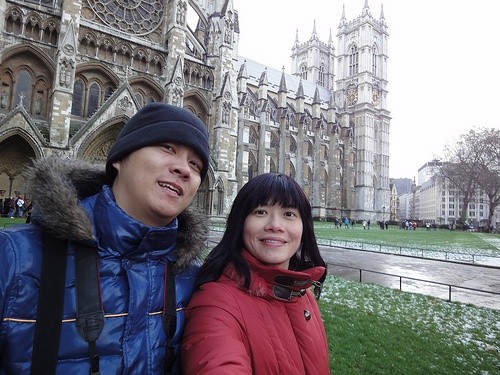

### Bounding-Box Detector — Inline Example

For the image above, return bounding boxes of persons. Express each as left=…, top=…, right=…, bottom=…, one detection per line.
left=334, top=217, right=494, bottom=233
left=0, top=195, right=32, bottom=223
left=0, top=103, right=210, bottom=375
left=181, top=172, right=330, bottom=375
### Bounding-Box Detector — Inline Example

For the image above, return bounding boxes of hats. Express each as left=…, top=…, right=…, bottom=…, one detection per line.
left=106, top=101, right=211, bottom=186
